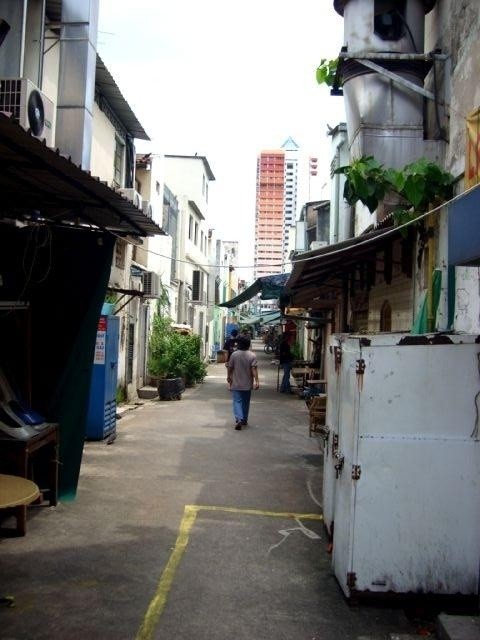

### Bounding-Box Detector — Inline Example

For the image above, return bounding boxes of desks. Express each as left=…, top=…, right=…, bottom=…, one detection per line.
left=0, top=422, right=62, bottom=537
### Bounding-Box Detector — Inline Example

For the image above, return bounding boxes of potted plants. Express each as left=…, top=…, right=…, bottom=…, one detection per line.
left=147, top=310, right=202, bottom=401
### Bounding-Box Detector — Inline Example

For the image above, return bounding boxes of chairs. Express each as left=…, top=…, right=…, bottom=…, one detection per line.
left=305, top=394, right=326, bottom=437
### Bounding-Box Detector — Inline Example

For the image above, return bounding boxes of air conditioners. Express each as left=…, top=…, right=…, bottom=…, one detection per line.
left=0, top=77, right=57, bottom=148
left=142, top=271, right=160, bottom=298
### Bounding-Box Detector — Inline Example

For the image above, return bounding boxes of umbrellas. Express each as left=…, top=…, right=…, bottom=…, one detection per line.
left=411, top=266, right=444, bottom=335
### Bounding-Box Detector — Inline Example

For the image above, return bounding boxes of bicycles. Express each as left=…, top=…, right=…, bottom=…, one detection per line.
left=264, top=338, right=279, bottom=354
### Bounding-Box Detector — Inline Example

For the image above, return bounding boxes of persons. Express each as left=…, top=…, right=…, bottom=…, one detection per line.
left=223, top=328, right=240, bottom=390
left=307, top=336, right=322, bottom=380
left=226, top=335, right=260, bottom=431
left=277, top=329, right=297, bottom=394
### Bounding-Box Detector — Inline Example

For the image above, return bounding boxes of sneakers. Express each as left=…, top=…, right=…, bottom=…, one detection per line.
left=236, top=418, right=249, bottom=431
